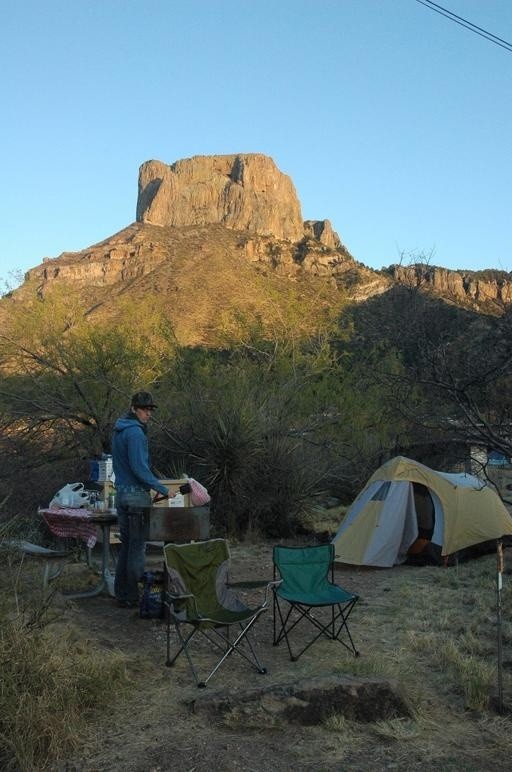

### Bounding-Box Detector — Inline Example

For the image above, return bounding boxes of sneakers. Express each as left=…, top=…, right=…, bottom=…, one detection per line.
left=119, top=598, right=141, bottom=609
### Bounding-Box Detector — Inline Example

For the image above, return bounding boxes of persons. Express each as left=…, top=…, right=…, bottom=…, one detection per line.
left=110, top=391, right=177, bottom=609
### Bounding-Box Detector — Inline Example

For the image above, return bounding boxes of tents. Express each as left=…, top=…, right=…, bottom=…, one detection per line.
left=330, top=455, right=512, bottom=568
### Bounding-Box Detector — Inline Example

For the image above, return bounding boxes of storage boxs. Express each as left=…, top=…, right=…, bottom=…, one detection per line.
left=88, top=459, right=194, bottom=544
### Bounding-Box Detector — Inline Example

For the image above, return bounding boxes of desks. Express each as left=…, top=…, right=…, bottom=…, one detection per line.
left=38, top=508, right=119, bottom=600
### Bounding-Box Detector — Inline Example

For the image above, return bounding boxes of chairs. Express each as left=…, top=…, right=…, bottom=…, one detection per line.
left=163, top=539, right=282, bottom=688
left=271, top=544, right=360, bottom=662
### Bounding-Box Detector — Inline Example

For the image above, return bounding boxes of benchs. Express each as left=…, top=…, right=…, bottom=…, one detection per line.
left=2, top=538, right=69, bottom=599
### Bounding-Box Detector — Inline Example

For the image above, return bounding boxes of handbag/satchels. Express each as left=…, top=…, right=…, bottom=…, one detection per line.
left=142, top=570, right=167, bottom=619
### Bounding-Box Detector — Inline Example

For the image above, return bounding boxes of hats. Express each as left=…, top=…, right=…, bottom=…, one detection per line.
left=131, top=392, right=158, bottom=408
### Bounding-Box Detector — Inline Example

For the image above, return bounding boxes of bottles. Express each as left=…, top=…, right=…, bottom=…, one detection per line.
left=109, top=488, right=116, bottom=508
